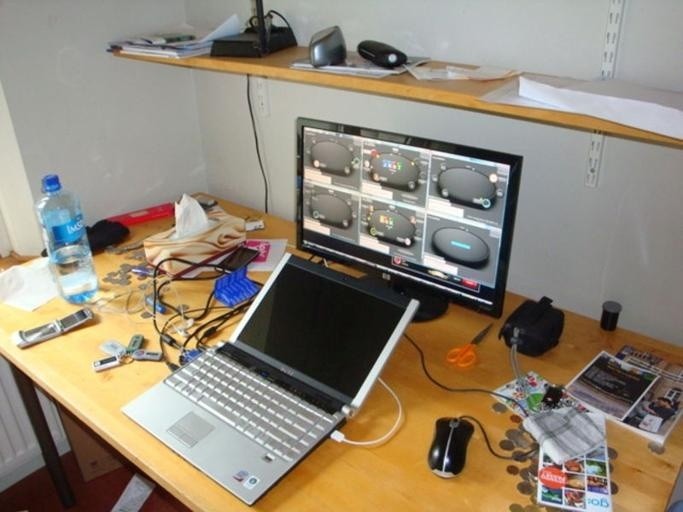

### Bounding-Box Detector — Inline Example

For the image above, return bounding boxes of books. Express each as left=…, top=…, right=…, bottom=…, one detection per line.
left=490, top=341, right=683, bottom=512
left=292, top=49, right=432, bottom=76
left=110, top=13, right=247, bottom=61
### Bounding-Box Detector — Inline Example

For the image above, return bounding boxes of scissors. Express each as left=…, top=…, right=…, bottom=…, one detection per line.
left=446, top=324, right=493, bottom=367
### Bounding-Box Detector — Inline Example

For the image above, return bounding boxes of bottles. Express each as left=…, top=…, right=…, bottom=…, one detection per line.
left=601, top=300, right=622, bottom=330
left=33, top=173, right=99, bottom=301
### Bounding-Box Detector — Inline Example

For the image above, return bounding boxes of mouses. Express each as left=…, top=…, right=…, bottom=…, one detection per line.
left=428, top=417, right=473, bottom=478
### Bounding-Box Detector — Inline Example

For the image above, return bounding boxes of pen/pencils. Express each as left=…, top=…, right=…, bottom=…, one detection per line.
left=146, top=296, right=166, bottom=314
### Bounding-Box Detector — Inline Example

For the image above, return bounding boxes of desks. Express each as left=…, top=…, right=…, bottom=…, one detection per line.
left=0, top=191, right=683, bottom=511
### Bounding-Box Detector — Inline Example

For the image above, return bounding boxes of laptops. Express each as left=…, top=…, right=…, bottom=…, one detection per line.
left=120, top=252, right=420, bottom=507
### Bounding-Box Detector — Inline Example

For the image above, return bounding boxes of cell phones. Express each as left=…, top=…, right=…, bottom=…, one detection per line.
left=215, top=245, right=259, bottom=275
left=15, top=307, right=94, bottom=349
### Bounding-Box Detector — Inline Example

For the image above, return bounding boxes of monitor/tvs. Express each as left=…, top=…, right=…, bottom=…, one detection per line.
left=296, top=116, right=522, bottom=322
